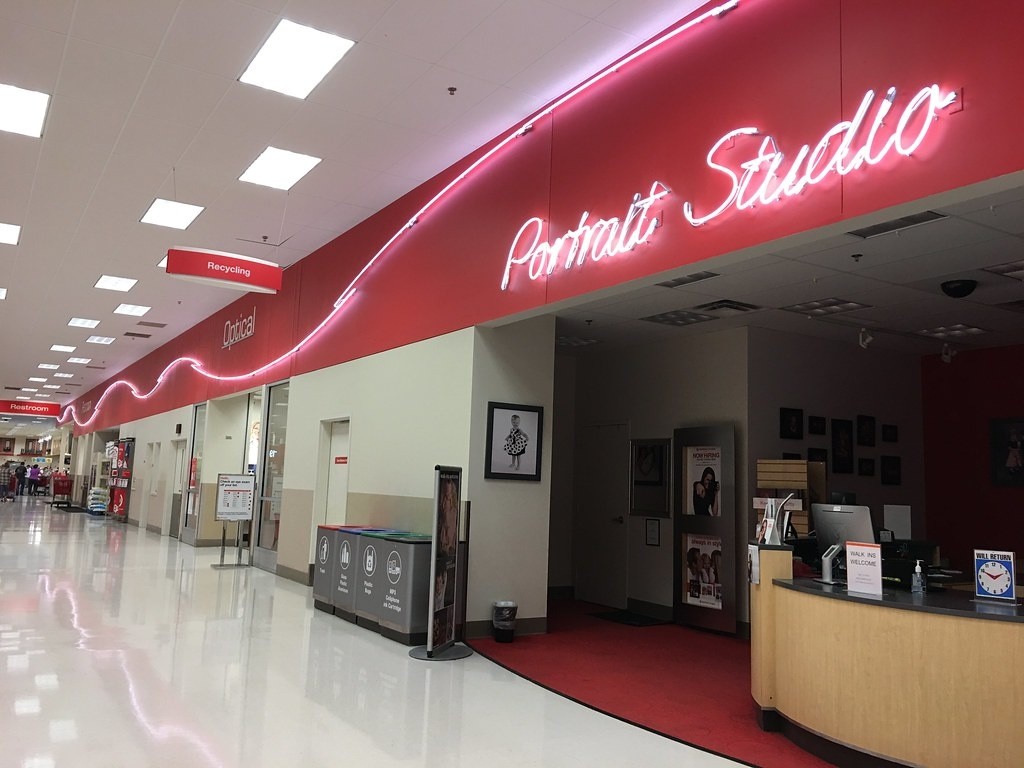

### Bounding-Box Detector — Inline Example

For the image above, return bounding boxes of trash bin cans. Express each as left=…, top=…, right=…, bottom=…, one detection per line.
left=492, top=600, right=519, bottom=644
left=354, top=532, right=410, bottom=634
left=334, top=528, right=409, bottom=625
left=313, top=524, right=384, bottom=616
left=380, top=537, right=467, bottom=647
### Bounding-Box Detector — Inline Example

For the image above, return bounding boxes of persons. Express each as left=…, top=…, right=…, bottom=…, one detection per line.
left=28, top=464, right=42, bottom=496
left=693, top=467, right=718, bottom=516
left=504, top=415, right=529, bottom=470
left=15, top=462, right=27, bottom=495
left=25, top=464, right=32, bottom=488
left=0, top=463, right=11, bottom=502
left=432, top=477, right=459, bottom=611
left=687, top=548, right=721, bottom=599
left=248, top=422, right=261, bottom=476
left=37, top=466, right=70, bottom=478
left=757, top=520, right=767, bottom=544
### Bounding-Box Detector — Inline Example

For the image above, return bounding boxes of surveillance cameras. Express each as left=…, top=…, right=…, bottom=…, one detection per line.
left=942, top=279, right=978, bottom=298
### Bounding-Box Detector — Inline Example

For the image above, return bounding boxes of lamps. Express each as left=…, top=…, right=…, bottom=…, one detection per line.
left=856, top=332, right=875, bottom=352
left=942, top=343, right=958, bottom=363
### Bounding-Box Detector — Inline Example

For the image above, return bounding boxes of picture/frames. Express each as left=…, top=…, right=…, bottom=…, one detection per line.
left=880, top=455, right=901, bottom=486
left=989, top=417, right=1024, bottom=487
left=830, top=418, right=854, bottom=474
left=882, top=424, right=898, bottom=443
left=858, top=457, right=875, bottom=476
left=808, top=415, right=826, bottom=435
left=807, top=447, right=828, bottom=481
left=631, top=437, right=673, bottom=518
left=635, top=445, right=664, bottom=485
left=857, top=415, right=876, bottom=447
left=782, top=453, right=802, bottom=461
left=484, top=402, right=543, bottom=482
left=780, top=407, right=803, bottom=440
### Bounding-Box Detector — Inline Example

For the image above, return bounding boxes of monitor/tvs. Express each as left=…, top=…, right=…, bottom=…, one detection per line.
left=811, top=504, right=875, bottom=585
